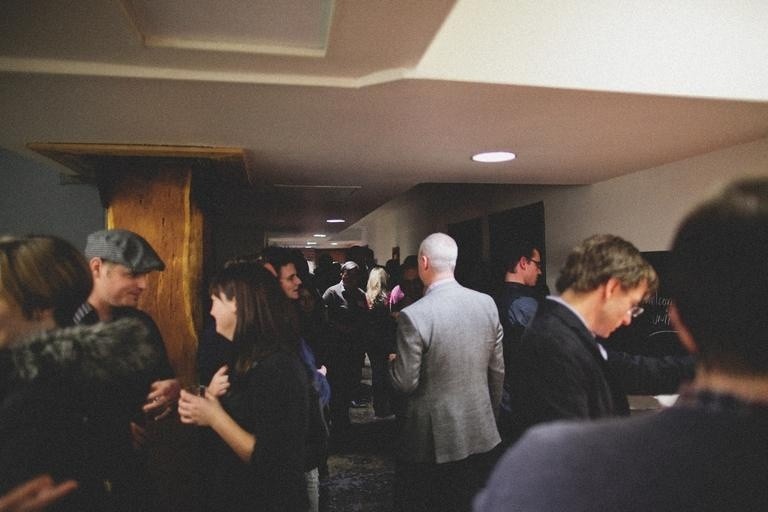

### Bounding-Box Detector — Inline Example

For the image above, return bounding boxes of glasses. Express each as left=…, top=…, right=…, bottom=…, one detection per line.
left=624, top=286, right=645, bottom=318
left=527, top=257, right=541, bottom=269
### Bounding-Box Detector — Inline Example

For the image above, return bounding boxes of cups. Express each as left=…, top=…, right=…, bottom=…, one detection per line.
left=184, top=384, right=209, bottom=402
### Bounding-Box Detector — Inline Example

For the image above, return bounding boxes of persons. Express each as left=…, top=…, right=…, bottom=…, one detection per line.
left=178, top=232, right=505, bottom=512
left=1, top=230, right=184, bottom=512
left=472, top=176, right=768, bottom=512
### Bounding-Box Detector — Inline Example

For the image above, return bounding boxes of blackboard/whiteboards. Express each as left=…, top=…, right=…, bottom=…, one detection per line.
left=596, top=250, right=691, bottom=359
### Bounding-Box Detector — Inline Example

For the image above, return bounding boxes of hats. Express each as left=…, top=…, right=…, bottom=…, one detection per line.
left=340, top=261, right=360, bottom=271
left=84, top=229, right=165, bottom=273
